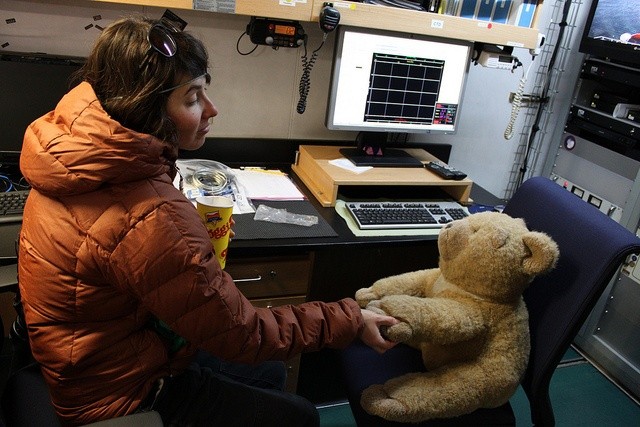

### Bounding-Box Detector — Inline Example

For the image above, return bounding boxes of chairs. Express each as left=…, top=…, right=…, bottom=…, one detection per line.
left=340, top=175, right=640, bottom=426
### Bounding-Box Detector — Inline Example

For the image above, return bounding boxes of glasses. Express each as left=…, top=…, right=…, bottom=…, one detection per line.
left=138, top=17, right=182, bottom=70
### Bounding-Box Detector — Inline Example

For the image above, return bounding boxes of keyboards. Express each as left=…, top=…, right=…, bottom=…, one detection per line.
left=345, top=200, right=470, bottom=229
left=0, top=190, right=30, bottom=223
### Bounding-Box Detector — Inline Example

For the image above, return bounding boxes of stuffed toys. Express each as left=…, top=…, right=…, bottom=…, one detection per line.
left=354, top=211, right=561, bottom=423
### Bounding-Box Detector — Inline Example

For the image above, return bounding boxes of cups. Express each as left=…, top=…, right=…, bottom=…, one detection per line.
left=195, top=195, right=233, bottom=269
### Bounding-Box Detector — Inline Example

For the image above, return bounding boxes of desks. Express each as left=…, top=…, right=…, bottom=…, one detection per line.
left=175, top=156, right=508, bottom=249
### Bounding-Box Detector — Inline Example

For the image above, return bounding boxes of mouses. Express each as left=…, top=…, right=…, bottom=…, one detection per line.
left=493, top=205, right=505, bottom=211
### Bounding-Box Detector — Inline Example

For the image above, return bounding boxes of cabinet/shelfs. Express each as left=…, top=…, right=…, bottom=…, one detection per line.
left=105, top=0, right=540, bottom=50
left=222, top=250, right=316, bottom=397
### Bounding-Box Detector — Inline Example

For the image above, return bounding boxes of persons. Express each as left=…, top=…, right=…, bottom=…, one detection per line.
left=17, top=16, right=402, bottom=425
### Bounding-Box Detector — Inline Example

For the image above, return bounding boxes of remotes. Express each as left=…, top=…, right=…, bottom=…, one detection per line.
left=424, top=162, right=456, bottom=180
left=432, top=160, right=467, bottom=180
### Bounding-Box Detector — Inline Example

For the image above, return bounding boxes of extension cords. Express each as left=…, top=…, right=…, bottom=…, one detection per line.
left=613, top=103, right=640, bottom=118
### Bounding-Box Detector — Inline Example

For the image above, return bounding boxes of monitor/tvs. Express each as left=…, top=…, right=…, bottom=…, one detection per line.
left=0, top=50, right=89, bottom=157
left=578, top=0, right=639, bottom=64
left=325, top=25, right=474, bottom=167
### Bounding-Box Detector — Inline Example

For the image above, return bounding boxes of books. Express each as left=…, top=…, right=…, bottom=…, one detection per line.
left=359, top=0, right=448, bottom=15
